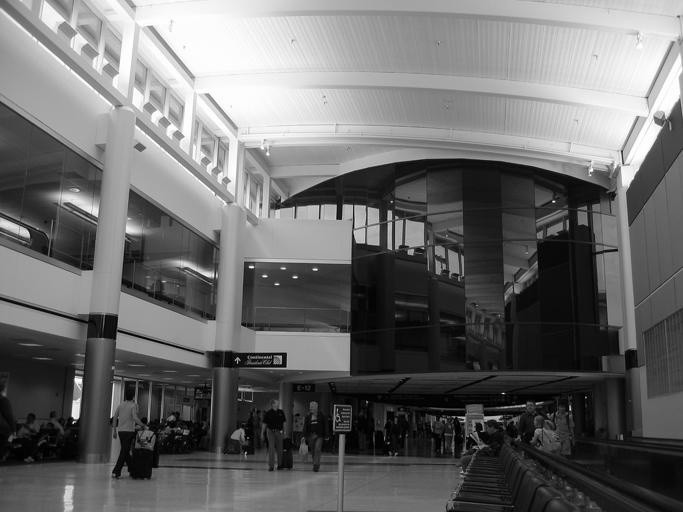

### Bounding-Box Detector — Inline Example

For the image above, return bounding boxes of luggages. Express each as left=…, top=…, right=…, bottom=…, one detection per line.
left=130, top=447, right=154, bottom=480
left=280, top=440, right=294, bottom=468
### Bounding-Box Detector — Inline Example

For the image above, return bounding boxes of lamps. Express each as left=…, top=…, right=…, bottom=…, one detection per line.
left=652, top=109, right=672, bottom=132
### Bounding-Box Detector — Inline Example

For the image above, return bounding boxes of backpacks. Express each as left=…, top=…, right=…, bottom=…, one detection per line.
left=134, top=430, right=155, bottom=450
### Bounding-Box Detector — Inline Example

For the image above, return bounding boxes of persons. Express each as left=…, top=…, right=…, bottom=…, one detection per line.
left=0, top=411, right=80, bottom=463
left=346, top=398, right=576, bottom=472
left=142, top=410, right=209, bottom=455
left=302, top=400, right=330, bottom=472
left=260, top=399, right=287, bottom=472
left=111, top=389, right=149, bottom=479
left=0, top=384, right=17, bottom=462
left=227, top=423, right=250, bottom=455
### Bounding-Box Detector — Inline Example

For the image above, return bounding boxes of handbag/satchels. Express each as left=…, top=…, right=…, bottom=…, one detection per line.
left=299, top=437, right=309, bottom=457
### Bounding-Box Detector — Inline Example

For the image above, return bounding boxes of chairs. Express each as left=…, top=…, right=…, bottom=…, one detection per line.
left=443, top=430, right=682, bottom=511
left=0, top=419, right=79, bottom=461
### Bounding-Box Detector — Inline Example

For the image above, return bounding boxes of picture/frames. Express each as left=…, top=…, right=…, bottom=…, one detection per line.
left=0, top=371, right=9, bottom=398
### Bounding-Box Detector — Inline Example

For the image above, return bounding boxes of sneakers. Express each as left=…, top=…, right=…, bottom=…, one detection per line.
left=455, top=461, right=469, bottom=478
left=268, top=466, right=283, bottom=471
left=110, top=472, right=123, bottom=480
left=387, top=450, right=400, bottom=458
left=312, top=465, right=320, bottom=472
left=23, top=456, right=34, bottom=464
